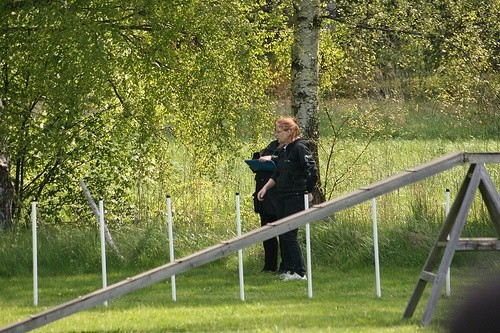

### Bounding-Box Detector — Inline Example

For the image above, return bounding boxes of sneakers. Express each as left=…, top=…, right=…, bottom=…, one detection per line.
left=279, top=270, right=291, bottom=280
left=281, top=271, right=308, bottom=282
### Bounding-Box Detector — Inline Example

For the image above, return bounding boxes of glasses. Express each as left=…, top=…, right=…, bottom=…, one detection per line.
left=275, top=129, right=287, bottom=133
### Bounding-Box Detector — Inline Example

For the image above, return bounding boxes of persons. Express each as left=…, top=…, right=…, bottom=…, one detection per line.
left=258, top=117, right=319, bottom=282
left=245, top=119, right=290, bottom=275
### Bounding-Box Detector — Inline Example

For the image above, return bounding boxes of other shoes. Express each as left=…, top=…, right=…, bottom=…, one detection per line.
left=260, top=269, right=278, bottom=273
